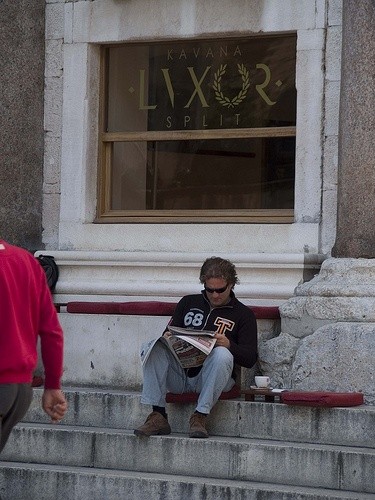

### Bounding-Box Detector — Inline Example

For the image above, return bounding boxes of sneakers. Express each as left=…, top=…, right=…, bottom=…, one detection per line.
left=134, top=412, right=171, bottom=436
left=190, top=413, right=209, bottom=438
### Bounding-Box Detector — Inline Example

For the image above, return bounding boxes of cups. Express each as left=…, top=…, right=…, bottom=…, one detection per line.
left=255, top=375, right=273, bottom=388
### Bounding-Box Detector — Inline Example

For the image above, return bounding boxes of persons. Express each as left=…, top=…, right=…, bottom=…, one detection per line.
left=0, top=237, right=68, bottom=455
left=133, top=256, right=259, bottom=440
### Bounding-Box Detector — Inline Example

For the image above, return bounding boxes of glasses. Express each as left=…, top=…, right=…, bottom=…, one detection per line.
left=204, top=282, right=229, bottom=293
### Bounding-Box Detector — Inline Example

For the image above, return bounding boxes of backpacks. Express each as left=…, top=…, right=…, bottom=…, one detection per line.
left=34, top=255, right=59, bottom=295
